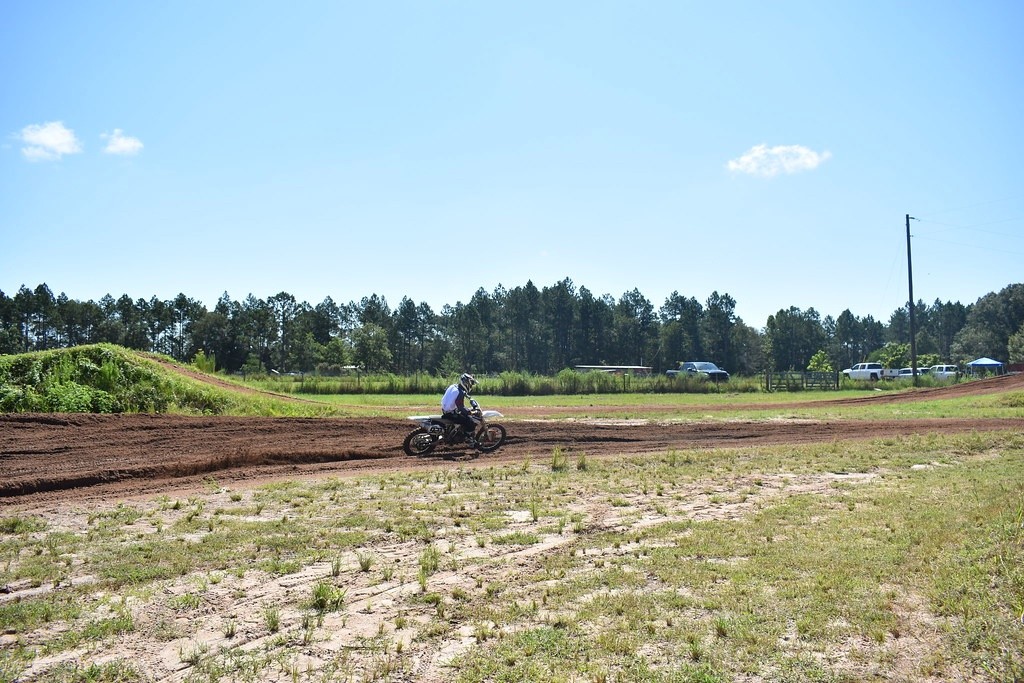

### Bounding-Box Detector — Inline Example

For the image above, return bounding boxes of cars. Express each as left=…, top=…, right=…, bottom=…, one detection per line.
left=899, top=368, right=922, bottom=378
left=930, top=365, right=959, bottom=379
left=918, top=367, right=930, bottom=375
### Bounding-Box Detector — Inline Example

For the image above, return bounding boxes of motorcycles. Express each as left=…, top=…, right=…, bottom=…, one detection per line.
left=403, top=393, right=506, bottom=456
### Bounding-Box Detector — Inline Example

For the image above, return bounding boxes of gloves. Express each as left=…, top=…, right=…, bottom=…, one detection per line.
left=476, top=410, right=481, bottom=416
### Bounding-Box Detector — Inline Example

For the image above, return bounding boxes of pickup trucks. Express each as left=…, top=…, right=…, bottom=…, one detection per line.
left=843, top=363, right=899, bottom=381
left=665, top=362, right=729, bottom=384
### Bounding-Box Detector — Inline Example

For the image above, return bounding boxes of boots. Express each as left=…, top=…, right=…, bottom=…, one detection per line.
left=465, top=431, right=477, bottom=444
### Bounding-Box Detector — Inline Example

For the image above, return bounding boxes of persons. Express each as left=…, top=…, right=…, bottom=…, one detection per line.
left=441, top=373, right=480, bottom=448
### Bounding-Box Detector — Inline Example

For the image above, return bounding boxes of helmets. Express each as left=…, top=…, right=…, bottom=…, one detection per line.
left=459, top=373, right=479, bottom=394
